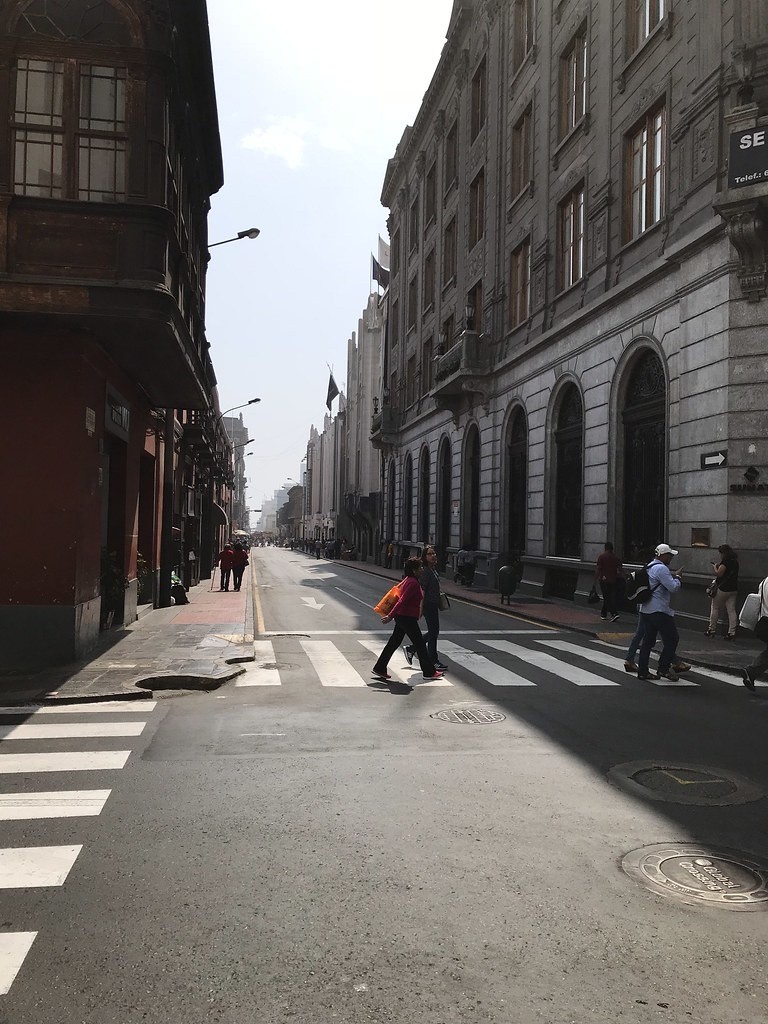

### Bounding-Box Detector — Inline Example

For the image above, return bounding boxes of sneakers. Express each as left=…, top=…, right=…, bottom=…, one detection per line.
left=403, top=645, right=415, bottom=665
left=433, top=661, right=448, bottom=670
left=371, top=668, right=391, bottom=679
left=422, top=671, right=443, bottom=679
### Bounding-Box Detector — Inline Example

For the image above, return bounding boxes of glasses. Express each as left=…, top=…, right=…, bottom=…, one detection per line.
left=427, top=553, right=437, bottom=556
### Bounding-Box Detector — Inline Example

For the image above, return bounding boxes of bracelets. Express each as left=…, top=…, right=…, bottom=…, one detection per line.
left=676, top=574, right=682, bottom=579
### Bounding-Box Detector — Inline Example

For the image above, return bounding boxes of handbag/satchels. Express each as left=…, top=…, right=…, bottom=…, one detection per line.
left=706, top=580, right=717, bottom=598
left=739, top=594, right=761, bottom=631
left=243, top=559, right=250, bottom=567
left=374, top=586, right=398, bottom=621
left=755, top=616, right=768, bottom=643
left=438, top=593, right=451, bottom=612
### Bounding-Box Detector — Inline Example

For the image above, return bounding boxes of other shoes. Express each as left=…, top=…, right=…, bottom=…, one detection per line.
left=657, top=670, right=679, bottom=682
left=704, top=629, right=715, bottom=638
left=638, top=672, right=660, bottom=680
left=624, top=661, right=639, bottom=672
left=601, top=615, right=607, bottom=620
left=608, top=613, right=620, bottom=624
left=741, top=668, right=756, bottom=691
left=672, top=663, right=691, bottom=672
left=724, top=633, right=736, bottom=642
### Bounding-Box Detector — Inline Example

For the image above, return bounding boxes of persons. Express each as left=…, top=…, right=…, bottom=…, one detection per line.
left=384, top=544, right=394, bottom=569
left=704, top=545, right=739, bottom=640
left=372, top=545, right=448, bottom=680
left=624, top=542, right=692, bottom=681
left=591, top=541, right=622, bottom=624
left=273, top=535, right=347, bottom=559
left=216, top=534, right=271, bottom=592
left=740, top=576, right=768, bottom=690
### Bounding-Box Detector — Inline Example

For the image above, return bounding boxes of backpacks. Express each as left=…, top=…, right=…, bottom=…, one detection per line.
left=626, top=563, right=663, bottom=604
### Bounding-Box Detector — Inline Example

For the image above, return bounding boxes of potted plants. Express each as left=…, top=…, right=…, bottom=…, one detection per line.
left=97, top=566, right=130, bottom=629
left=136, top=551, right=147, bottom=603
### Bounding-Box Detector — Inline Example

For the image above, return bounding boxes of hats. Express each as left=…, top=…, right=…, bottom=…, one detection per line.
left=655, top=544, right=678, bottom=555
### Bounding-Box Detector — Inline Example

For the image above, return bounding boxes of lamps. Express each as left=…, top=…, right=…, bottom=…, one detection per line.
left=235, top=452, right=253, bottom=465
left=216, top=398, right=260, bottom=436
left=282, top=486, right=290, bottom=491
left=731, top=44, right=758, bottom=107
left=207, top=228, right=260, bottom=247
left=436, top=331, right=447, bottom=355
left=465, top=304, right=476, bottom=331
left=373, top=396, right=379, bottom=414
left=287, top=477, right=299, bottom=486
left=381, top=387, right=390, bottom=404
left=224, top=439, right=254, bottom=456
